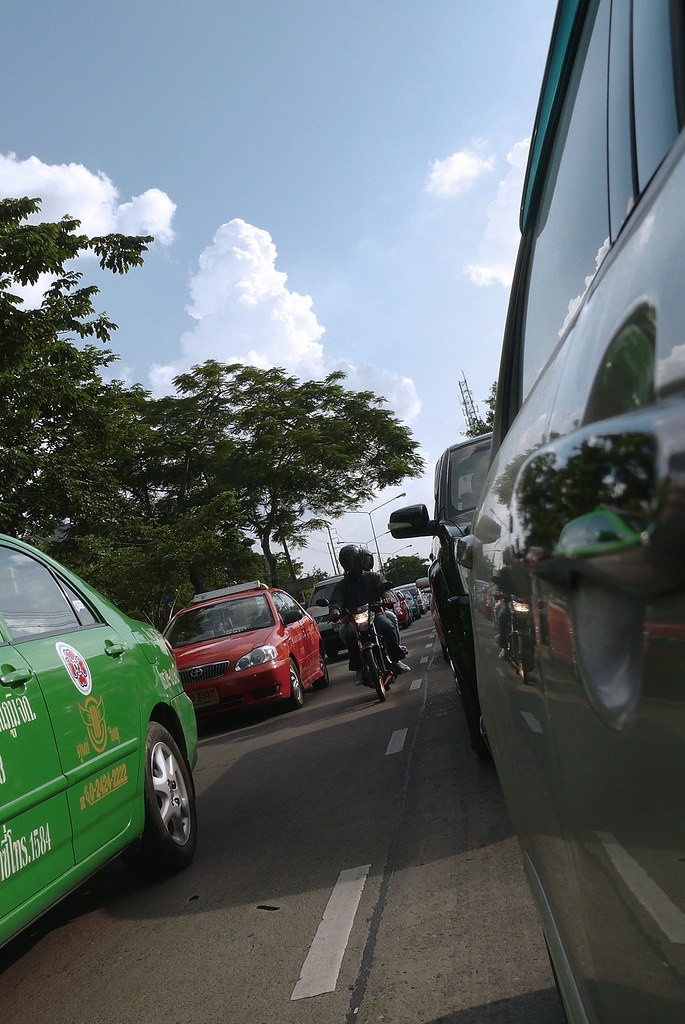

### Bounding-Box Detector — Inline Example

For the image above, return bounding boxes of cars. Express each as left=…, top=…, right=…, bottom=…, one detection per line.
left=157, top=579, right=330, bottom=710
left=0, top=533, right=198, bottom=951
left=306, top=571, right=430, bottom=651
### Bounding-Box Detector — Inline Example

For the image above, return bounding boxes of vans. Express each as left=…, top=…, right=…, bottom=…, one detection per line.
left=388, top=1, right=685, bottom=1022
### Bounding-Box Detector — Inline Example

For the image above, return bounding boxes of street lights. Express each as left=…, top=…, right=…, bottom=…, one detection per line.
left=329, top=492, right=413, bottom=576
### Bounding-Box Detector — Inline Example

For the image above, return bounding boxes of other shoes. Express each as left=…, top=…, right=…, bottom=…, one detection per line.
left=353, top=669, right=364, bottom=687
left=398, top=644, right=408, bottom=655
left=394, top=660, right=411, bottom=673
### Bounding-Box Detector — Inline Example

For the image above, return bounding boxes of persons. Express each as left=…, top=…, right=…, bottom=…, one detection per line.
left=494, top=547, right=537, bottom=658
left=329, top=545, right=411, bottom=685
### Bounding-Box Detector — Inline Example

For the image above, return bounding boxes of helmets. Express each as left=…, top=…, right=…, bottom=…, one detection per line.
left=340, top=546, right=359, bottom=570
left=358, top=548, right=374, bottom=571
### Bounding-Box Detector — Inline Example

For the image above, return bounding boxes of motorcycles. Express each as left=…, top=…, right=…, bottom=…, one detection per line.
left=315, top=582, right=407, bottom=702
